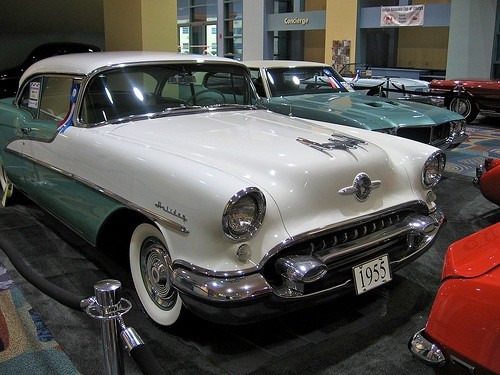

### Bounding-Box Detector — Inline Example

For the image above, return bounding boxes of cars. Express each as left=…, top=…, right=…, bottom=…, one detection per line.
left=428, top=77, right=500, bottom=124
left=307, top=62, right=438, bottom=105
left=0, top=50, right=448, bottom=338
left=179, top=60, right=471, bottom=153
left=408, top=160, right=500, bottom=375
